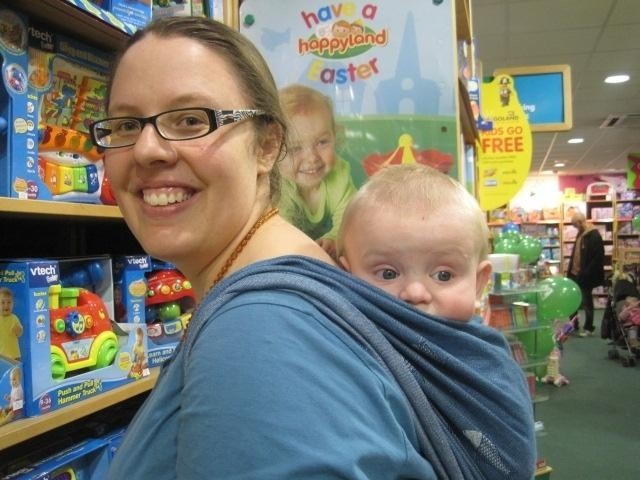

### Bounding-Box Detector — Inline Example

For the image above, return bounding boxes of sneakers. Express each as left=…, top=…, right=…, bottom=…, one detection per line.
left=577, top=327, right=596, bottom=338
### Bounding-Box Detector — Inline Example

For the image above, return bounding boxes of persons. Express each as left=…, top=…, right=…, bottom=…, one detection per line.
left=88, top=15, right=443, bottom=479
left=276, top=84, right=360, bottom=258
left=128, top=328, right=144, bottom=376
left=617, top=295, right=640, bottom=350
left=497, top=75, right=512, bottom=108
left=4, top=367, right=24, bottom=416
left=565, top=211, right=605, bottom=338
left=334, top=162, right=494, bottom=323
left=1, top=288, right=24, bottom=362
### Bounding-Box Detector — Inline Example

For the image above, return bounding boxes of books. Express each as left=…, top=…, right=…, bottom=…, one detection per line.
left=525, top=371, right=536, bottom=399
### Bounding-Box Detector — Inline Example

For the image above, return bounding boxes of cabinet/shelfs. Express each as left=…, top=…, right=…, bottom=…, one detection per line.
left=236, top=0, right=479, bottom=268
left=0, top=0, right=241, bottom=452
left=486, top=214, right=560, bottom=279
left=485, top=284, right=551, bottom=442
left=614, top=190, right=640, bottom=293
left=560, top=199, right=615, bottom=309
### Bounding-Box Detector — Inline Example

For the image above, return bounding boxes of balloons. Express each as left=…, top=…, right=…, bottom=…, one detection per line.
left=632, top=214, right=640, bottom=230
left=489, top=221, right=583, bottom=378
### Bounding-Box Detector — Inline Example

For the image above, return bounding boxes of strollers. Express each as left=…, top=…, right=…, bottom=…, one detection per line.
left=601, top=263, right=640, bottom=367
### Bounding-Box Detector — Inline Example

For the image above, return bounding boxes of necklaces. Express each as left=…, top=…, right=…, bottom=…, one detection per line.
left=179, top=207, right=279, bottom=348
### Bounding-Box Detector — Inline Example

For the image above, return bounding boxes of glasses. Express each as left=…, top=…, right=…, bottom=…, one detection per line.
left=87, top=105, right=272, bottom=149
